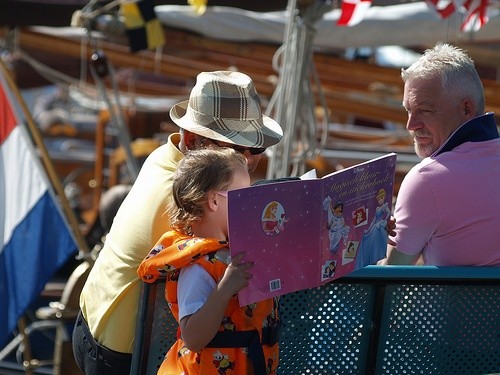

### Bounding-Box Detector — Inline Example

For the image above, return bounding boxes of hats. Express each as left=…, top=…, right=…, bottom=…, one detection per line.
left=170, top=70, right=283, bottom=148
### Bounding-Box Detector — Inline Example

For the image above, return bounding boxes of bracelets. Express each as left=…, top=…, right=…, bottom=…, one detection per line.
left=206, top=288, right=231, bottom=312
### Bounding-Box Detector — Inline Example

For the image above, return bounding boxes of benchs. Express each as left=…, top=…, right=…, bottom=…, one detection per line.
left=131, top=264, right=500, bottom=375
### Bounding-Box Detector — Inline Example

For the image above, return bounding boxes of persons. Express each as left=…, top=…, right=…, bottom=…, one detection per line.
left=2, top=183, right=132, bottom=369
left=137, top=147, right=397, bottom=375
left=377, top=44, right=500, bottom=267
left=72, top=71, right=283, bottom=375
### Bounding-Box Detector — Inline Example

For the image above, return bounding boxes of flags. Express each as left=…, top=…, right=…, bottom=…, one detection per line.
left=426, top=0, right=500, bottom=34
left=122, top=0, right=166, bottom=54
left=0, top=73, right=79, bottom=352
left=337, top=0, right=373, bottom=27
left=188, top=0, right=207, bottom=16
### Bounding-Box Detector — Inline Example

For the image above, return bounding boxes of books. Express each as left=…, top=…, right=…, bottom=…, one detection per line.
left=226, top=152, right=397, bottom=307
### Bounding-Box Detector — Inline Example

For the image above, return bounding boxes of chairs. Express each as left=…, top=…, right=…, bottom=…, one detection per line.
left=0, top=236, right=105, bottom=375
